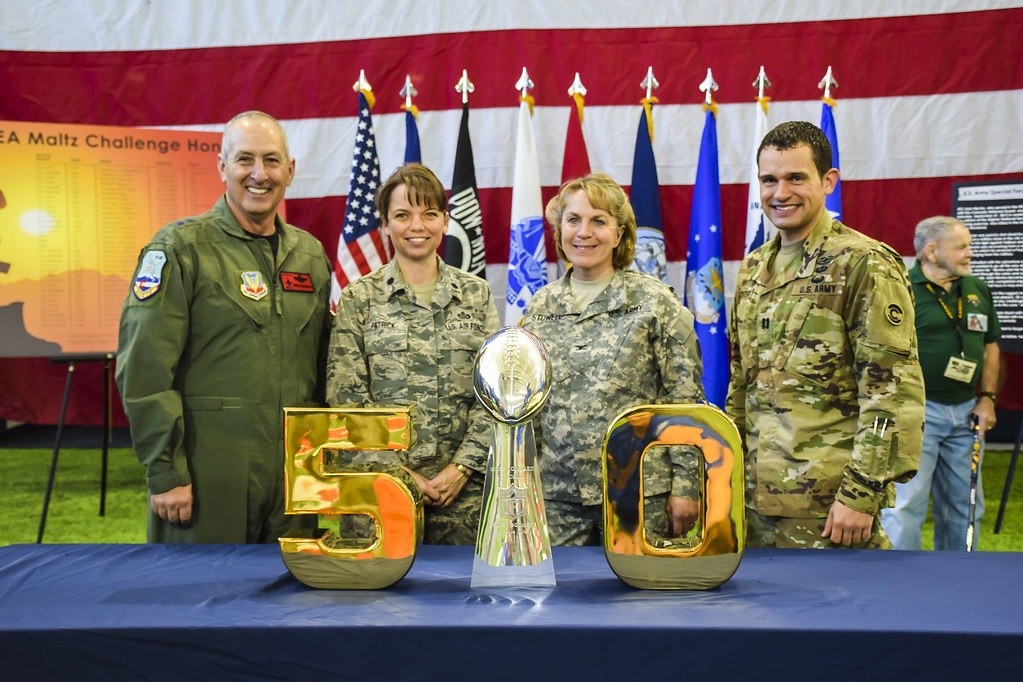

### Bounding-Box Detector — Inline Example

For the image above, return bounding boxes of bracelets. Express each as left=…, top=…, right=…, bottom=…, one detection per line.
left=977, top=390, right=998, bottom=405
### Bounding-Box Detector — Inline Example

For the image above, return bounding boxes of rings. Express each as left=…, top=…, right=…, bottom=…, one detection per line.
left=690, top=523, right=695, bottom=528
left=987, top=425, right=992, bottom=430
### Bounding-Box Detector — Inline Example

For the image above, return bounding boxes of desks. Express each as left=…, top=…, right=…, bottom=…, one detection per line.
left=0, top=542, right=1023, bottom=682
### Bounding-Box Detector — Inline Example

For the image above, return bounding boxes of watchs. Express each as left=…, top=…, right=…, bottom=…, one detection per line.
left=449, top=462, right=474, bottom=477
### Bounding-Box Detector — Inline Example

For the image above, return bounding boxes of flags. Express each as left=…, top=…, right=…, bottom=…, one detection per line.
left=328, top=88, right=844, bottom=414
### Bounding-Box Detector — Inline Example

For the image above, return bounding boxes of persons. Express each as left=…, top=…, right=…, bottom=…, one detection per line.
left=600, top=407, right=735, bottom=555
left=881, top=216, right=1001, bottom=555
left=325, top=162, right=503, bottom=545
left=718, top=120, right=925, bottom=553
left=512, top=170, right=708, bottom=553
left=117, top=109, right=331, bottom=543
